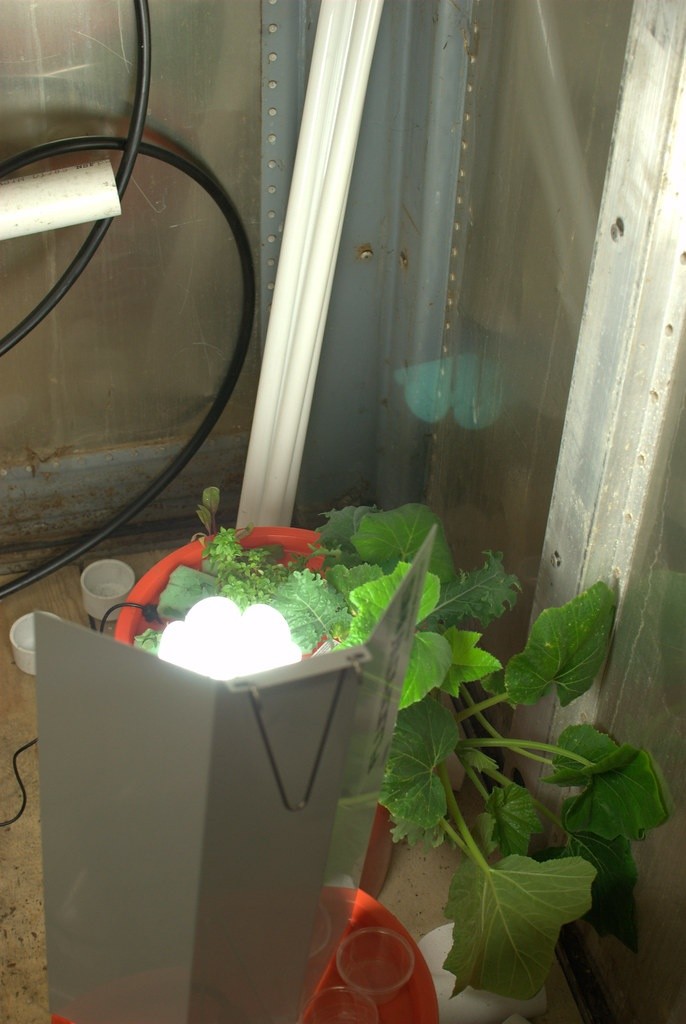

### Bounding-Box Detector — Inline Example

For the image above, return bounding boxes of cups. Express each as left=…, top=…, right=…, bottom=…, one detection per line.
left=334, top=926, right=413, bottom=1007
left=80, top=558, right=136, bottom=620
left=298, top=985, right=379, bottom=1024
left=9, top=610, right=60, bottom=676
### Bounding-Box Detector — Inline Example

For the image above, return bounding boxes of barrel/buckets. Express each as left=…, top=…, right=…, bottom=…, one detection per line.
left=294, top=887, right=441, bottom=1024
left=114, top=523, right=395, bottom=903
